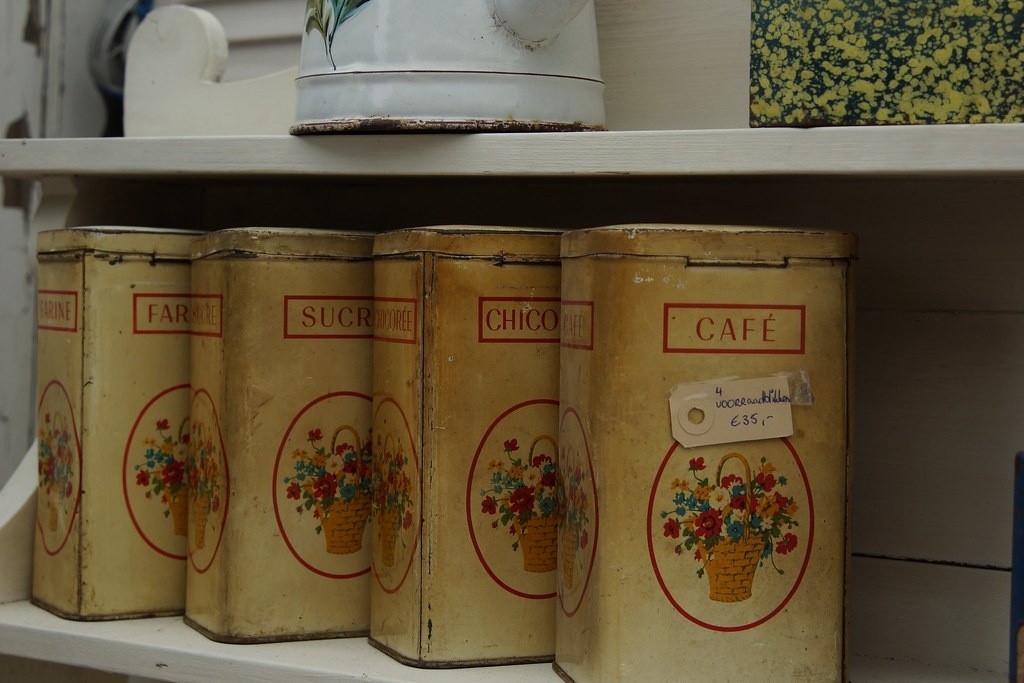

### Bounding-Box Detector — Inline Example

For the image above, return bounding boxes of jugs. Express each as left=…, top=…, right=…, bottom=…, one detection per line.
left=291, top=0, right=604, bottom=132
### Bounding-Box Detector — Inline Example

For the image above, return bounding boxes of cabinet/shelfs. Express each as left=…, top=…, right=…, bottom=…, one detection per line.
left=0, top=0, right=1024, bottom=683
